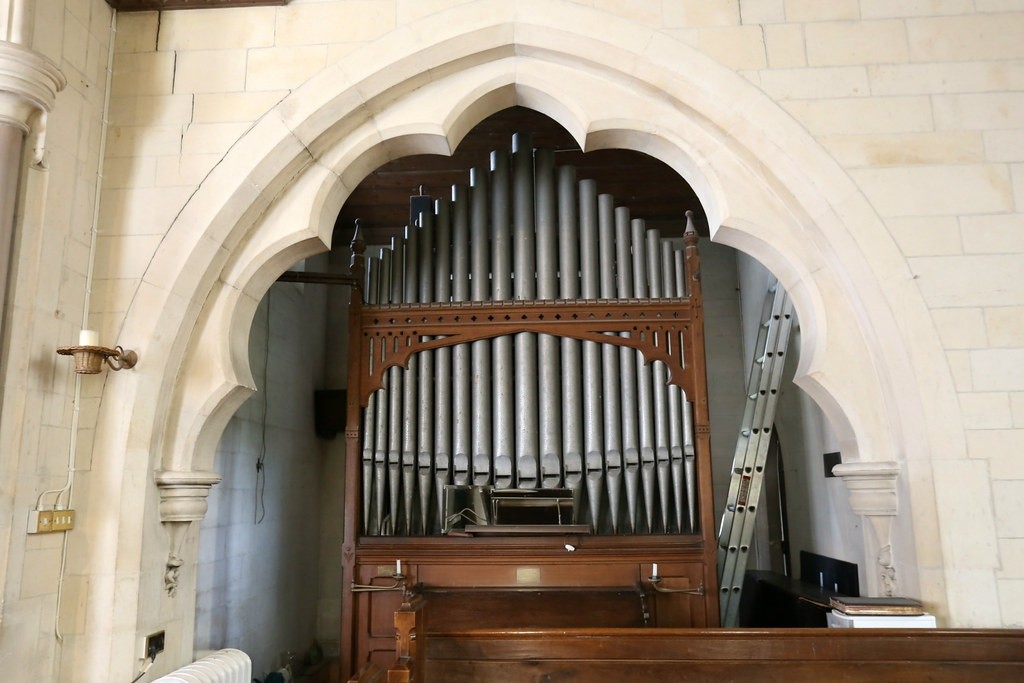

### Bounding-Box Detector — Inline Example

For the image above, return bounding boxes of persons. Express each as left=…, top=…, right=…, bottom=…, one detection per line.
left=829, top=595, right=925, bottom=616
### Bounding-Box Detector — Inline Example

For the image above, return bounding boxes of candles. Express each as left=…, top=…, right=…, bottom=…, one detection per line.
left=396, top=559, right=402, bottom=574
left=652, top=561, right=659, bottom=577
left=78, top=326, right=99, bottom=349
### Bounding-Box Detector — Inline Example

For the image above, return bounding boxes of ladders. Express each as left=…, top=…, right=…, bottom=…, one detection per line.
left=716, top=277, right=794, bottom=630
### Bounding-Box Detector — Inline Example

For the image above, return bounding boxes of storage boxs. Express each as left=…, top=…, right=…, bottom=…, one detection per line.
left=823, top=612, right=938, bottom=631
